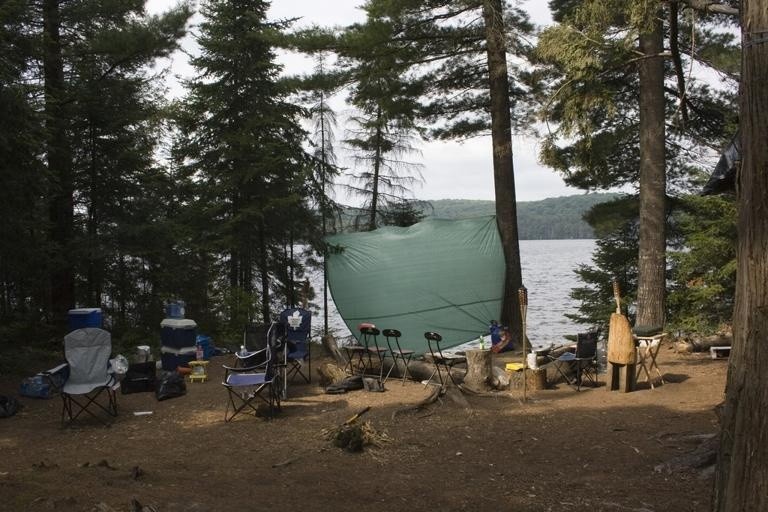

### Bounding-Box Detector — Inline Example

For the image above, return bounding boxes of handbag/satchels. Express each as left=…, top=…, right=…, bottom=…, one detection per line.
left=490, top=319, right=514, bottom=354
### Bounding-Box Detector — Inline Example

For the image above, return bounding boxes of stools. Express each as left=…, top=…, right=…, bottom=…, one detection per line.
left=188, top=361, right=209, bottom=384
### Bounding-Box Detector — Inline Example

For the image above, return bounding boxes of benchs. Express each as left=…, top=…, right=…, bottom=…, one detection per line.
left=709, top=339, right=731, bottom=359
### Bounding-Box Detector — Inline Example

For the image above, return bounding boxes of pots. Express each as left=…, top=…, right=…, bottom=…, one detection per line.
left=530, top=342, right=552, bottom=356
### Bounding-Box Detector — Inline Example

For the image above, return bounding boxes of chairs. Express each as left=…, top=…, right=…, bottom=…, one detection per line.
left=356, top=326, right=389, bottom=382
left=342, top=322, right=376, bottom=377
left=423, top=332, right=467, bottom=393
left=547, top=331, right=598, bottom=392
left=381, top=328, right=416, bottom=386
left=38, top=325, right=124, bottom=426
left=222, top=307, right=314, bottom=423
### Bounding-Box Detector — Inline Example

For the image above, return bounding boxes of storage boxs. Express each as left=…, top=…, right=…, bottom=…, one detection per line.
left=161, top=319, right=198, bottom=348
left=120, top=361, right=158, bottom=394
left=160, top=345, right=197, bottom=372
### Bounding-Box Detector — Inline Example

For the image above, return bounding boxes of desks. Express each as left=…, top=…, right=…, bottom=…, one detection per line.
left=630, top=330, right=669, bottom=391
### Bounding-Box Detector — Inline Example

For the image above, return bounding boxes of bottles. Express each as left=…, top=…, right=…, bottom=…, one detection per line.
left=240, top=345, right=248, bottom=356
left=479, top=335, right=484, bottom=350
left=196, top=344, right=204, bottom=360
